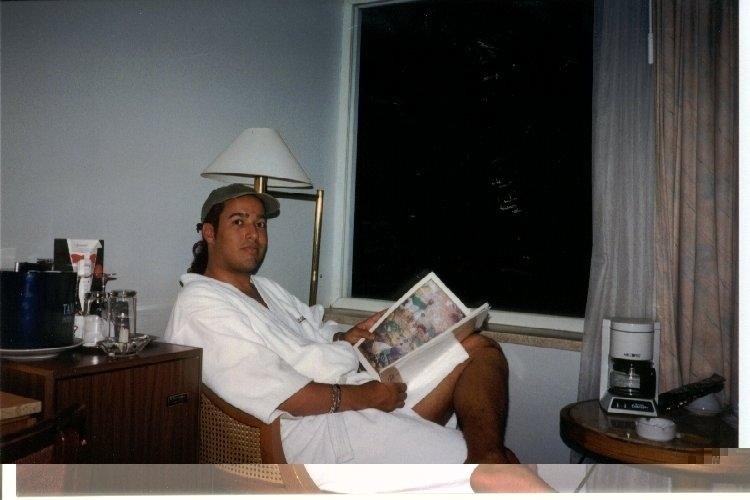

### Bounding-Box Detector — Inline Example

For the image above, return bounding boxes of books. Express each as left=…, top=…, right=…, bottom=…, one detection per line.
left=353, top=271, right=490, bottom=385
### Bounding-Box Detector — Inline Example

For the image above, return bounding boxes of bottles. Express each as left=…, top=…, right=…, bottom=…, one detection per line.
left=112, top=290, right=137, bottom=334
left=82, top=291, right=112, bottom=344
left=114, top=303, right=132, bottom=344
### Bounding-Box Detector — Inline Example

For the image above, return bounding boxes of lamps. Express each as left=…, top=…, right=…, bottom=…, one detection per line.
left=201, top=127, right=324, bottom=304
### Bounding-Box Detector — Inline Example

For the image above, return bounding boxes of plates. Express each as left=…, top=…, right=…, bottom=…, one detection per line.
left=685, top=407, right=725, bottom=418
left=1, top=337, right=85, bottom=363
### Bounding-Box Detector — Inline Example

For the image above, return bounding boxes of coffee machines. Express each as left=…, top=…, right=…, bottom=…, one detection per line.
left=596, top=315, right=662, bottom=418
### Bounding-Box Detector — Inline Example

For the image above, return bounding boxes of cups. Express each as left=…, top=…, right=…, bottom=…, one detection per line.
left=663, top=405, right=689, bottom=436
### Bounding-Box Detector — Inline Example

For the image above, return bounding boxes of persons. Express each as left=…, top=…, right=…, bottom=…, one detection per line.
left=165, top=182, right=521, bottom=465
left=303, top=465, right=559, bottom=493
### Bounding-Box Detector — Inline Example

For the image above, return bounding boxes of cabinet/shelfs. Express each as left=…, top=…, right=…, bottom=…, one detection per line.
left=0, top=340, right=203, bottom=495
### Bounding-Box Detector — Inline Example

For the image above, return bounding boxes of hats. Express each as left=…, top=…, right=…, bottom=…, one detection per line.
left=202, top=183, right=280, bottom=224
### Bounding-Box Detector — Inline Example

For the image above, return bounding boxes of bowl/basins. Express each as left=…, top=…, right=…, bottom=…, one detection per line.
left=97, top=334, right=151, bottom=359
left=687, top=392, right=722, bottom=411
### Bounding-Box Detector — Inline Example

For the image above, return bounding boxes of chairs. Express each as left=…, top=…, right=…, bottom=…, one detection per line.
left=201, top=380, right=325, bottom=494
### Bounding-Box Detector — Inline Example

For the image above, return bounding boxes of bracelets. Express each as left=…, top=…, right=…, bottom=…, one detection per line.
left=327, top=383, right=342, bottom=414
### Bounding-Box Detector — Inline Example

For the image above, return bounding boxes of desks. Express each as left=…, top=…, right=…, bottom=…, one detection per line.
left=559, top=399, right=738, bottom=494
